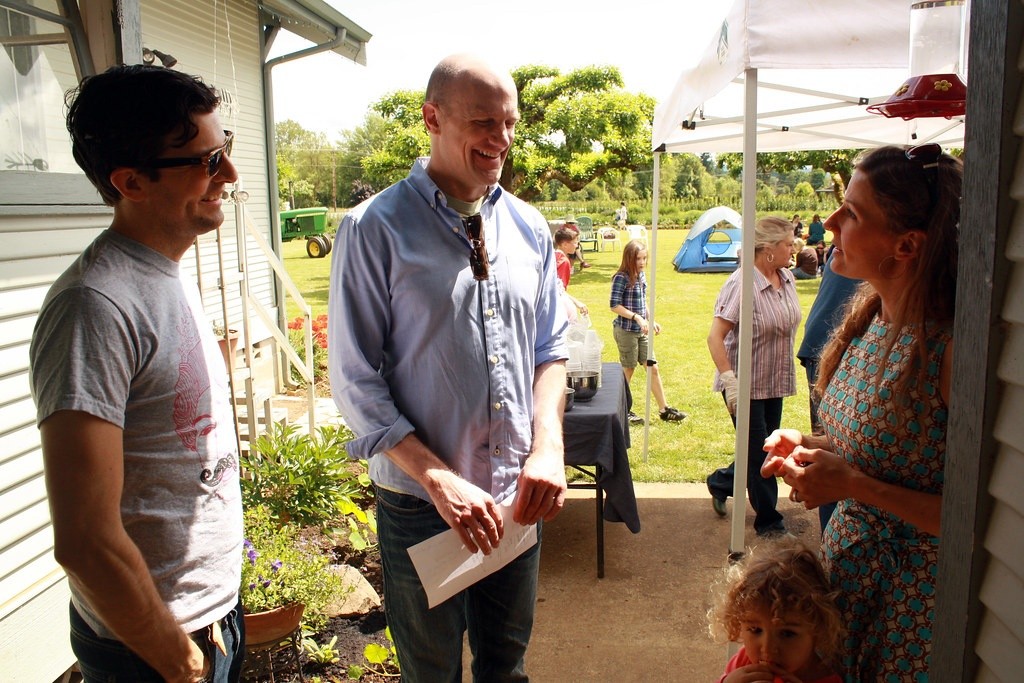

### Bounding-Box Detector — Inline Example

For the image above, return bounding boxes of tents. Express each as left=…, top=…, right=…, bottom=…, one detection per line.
left=641, top=0, right=970, bottom=565
left=670, top=206, right=742, bottom=273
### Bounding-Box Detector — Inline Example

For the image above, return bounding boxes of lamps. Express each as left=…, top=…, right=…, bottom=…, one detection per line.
left=144, top=47, right=177, bottom=67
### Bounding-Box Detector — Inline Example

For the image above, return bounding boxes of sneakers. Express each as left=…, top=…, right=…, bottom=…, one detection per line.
left=628, top=413, right=643, bottom=424
left=659, top=408, right=686, bottom=421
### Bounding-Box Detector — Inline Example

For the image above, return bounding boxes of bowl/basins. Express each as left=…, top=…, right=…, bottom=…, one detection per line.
left=566, top=370, right=599, bottom=402
left=564, top=388, right=575, bottom=411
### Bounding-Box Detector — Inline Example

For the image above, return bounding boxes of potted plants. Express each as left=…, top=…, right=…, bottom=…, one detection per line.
left=213, top=319, right=239, bottom=372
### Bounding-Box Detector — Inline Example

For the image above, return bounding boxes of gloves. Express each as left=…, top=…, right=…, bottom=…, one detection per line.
left=720, top=370, right=738, bottom=417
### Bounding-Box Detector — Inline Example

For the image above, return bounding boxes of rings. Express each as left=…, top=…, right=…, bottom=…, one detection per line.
left=793, top=490, right=800, bottom=504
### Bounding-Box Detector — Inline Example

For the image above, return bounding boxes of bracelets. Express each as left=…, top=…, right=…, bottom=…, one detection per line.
left=632, top=312, right=637, bottom=320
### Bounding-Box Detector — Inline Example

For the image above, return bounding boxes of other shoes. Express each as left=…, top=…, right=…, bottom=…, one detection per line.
left=580, top=261, right=590, bottom=268
left=764, top=528, right=798, bottom=541
left=713, top=496, right=727, bottom=516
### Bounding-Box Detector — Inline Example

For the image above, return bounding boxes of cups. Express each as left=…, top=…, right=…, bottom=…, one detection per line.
left=567, top=329, right=602, bottom=387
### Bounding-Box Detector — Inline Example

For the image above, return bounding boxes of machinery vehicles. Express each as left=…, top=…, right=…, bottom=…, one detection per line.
left=279, top=181, right=333, bottom=259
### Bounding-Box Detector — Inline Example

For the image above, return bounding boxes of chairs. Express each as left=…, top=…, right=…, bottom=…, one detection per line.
left=624, top=225, right=648, bottom=249
left=576, top=215, right=599, bottom=251
left=599, top=228, right=621, bottom=251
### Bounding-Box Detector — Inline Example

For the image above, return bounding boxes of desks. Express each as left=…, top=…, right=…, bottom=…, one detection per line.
left=563, top=363, right=641, bottom=579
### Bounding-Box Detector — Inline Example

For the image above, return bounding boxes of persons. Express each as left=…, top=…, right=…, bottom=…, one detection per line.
left=328, top=50, right=570, bottom=683
left=760, top=142, right=963, bottom=683
left=796, top=254, right=866, bottom=532
left=554, top=226, right=590, bottom=317
left=610, top=240, right=687, bottom=425
left=790, top=214, right=802, bottom=238
left=620, top=201, right=627, bottom=230
left=807, top=214, right=825, bottom=245
left=615, top=209, right=620, bottom=221
left=698, top=528, right=849, bottom=683
left=28, top=63, right=246, bottom=683
left=560, top=218, right=590, bottom=274
left=706, top=216, right=802, bottom=540
left=815, top=240, right=826, bottom=274
left=789, top=239, right=818, bottom=278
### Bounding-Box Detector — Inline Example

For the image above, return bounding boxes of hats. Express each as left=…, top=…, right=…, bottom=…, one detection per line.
left=564, top=214, right=578, bottom=223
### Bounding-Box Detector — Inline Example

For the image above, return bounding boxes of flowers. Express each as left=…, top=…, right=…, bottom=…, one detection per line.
left=241, top=532, right=311, bottom=612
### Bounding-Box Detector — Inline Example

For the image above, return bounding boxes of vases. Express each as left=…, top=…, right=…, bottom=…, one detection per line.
left=244, top=600, right=306, bottom=645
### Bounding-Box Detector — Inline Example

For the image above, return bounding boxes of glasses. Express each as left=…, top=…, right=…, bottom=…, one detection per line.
left=461, top=211, right=489, bottom=282
left=905, top=143, right=943, bottom=208
left=569, top=222, right=574, bottom=224
left=151, top=130, right=237, bottom=178
